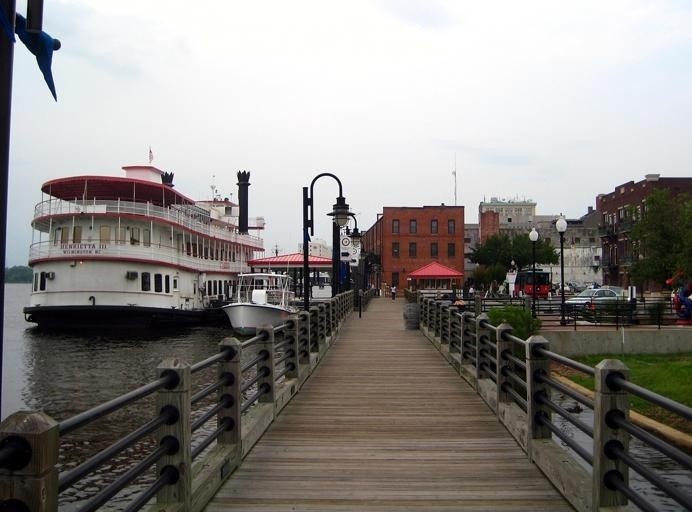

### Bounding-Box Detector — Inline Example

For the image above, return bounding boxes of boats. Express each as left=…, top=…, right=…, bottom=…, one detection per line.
left=297, top=271, right=332, bottom=299
left=22, top=165, right=265, bottom=331
left=221, top=269, right=300, bottom=336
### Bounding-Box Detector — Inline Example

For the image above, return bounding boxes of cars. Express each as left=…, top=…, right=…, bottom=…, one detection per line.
left=600, top=285, right=627, bottom=300
left=549, top=281, right=598, bottom=293
left=565, top=289, right=629, bottom=317
left=670, top=285, right=692, bottom=317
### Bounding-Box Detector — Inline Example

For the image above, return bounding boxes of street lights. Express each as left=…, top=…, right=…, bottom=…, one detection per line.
left=339, top=237, right=377, bottom=319
left=271, top=243, right=283, bottom=256
left=476, top=262, right=479, bottom=291
left=529, top=227, right=538, bottom=319
left=556, top=213, right=567, bottom=325
left=638, top=254, right=645, bottom=303
left=303, top=173, right=355, bottom=357
left=331, top=213, right=362, bottom=330
left=549, top=262, right=553, bottom=295
left=511, top=260, right=515, bottom=272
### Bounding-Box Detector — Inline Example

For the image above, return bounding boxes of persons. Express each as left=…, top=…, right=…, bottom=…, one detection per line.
left=390, top=283, right=397, bottom=302
left=555, top=283, right=560, bottom=296
left=464, top=280, right=509, bottom=299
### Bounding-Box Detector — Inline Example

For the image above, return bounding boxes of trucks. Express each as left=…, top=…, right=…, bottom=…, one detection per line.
left=505, top=269, right=549, bottom=301
